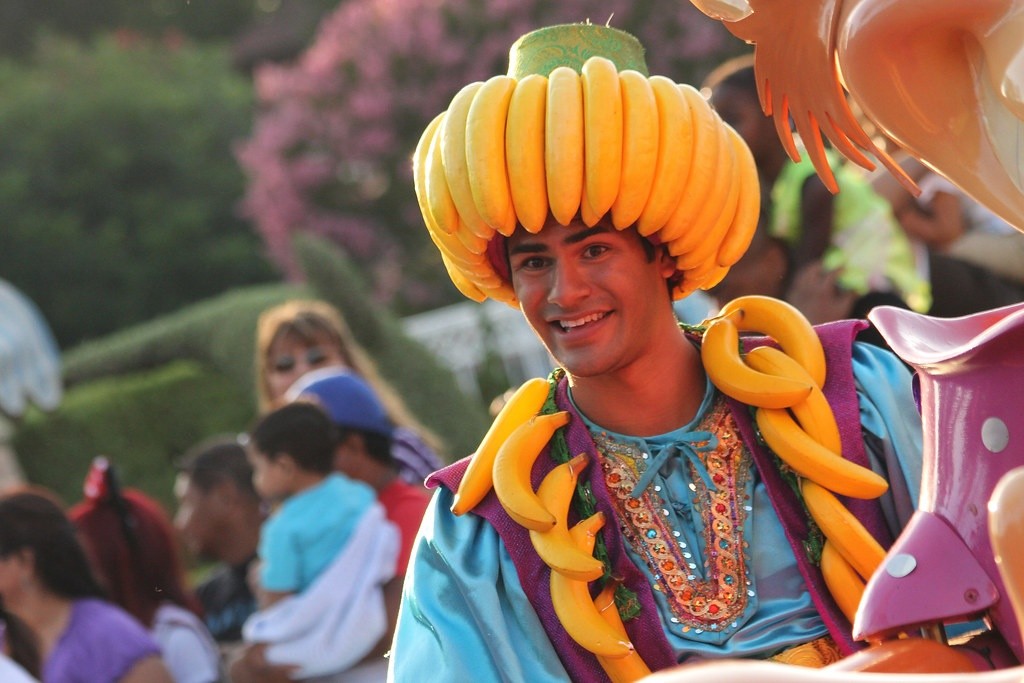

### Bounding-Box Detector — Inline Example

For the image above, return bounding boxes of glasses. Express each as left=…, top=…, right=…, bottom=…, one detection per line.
left=266, top=350, right=340, bottom=372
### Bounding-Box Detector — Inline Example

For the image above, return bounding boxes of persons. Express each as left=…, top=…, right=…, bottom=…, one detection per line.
left=675, top=38, right=1024, bottom=327
left=381, top=169, right=933, bottom=683
left=1, top=301, right=449, bottom=683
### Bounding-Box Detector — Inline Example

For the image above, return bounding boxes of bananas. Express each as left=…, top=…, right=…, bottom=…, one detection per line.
left=697, top=296, right=913, bottom=648
left=452, top=377, right=653, bottom=683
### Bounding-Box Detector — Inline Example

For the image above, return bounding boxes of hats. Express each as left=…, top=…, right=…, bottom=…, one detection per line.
left=293, top=371, right=394, bottom=437
left=409, top=22, right=762, bottom=301
